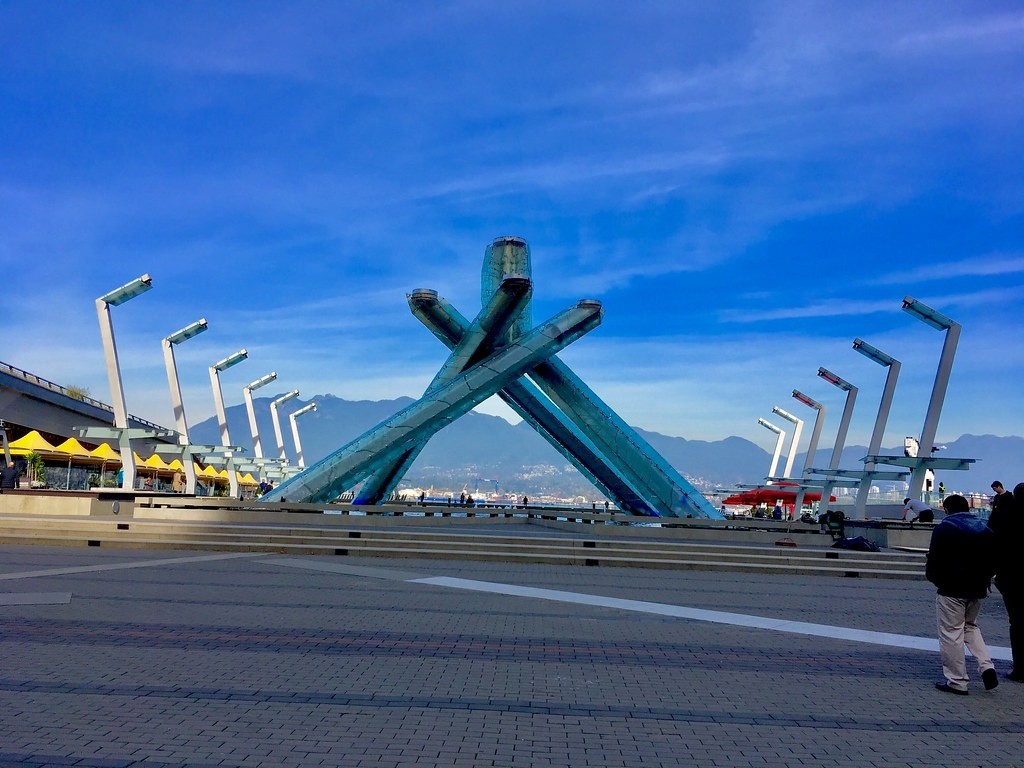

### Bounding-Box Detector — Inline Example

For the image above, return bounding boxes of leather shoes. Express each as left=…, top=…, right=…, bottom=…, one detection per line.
left=982, top=668, right=998, bottom=690
left=936, top=681, right=969, bottom=695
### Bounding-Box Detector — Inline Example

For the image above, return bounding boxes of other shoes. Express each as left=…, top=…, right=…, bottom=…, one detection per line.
left=1007, top=670, right=1024, bottom=683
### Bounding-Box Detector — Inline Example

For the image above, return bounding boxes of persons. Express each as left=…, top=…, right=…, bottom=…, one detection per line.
left=900, top=497, right=934, bottom=531
left=927, top=495, right=999, bottom=695
left=987, top=480, right=1024, bottom=684
left=420, top=492, right=424, bottom=502
left=258, top=479, right=274, bottom=495
left=750, top=505, right=758, bottom=517
left=605, top=501, right=609, bottom=509
left=171, top=468, right=182, bottom=493
left=0, top=462, right=21, bottom=489
left=938, top=482, right=946, bottom=503
left=460, top=493, right=465, bottom=500
left=523, top=495, right=528, bottom=505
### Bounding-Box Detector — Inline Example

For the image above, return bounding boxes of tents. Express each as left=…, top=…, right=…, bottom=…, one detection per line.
left=0, top=431, right=260, bottom=498
left=722, top=482, right=836, bottom=520
